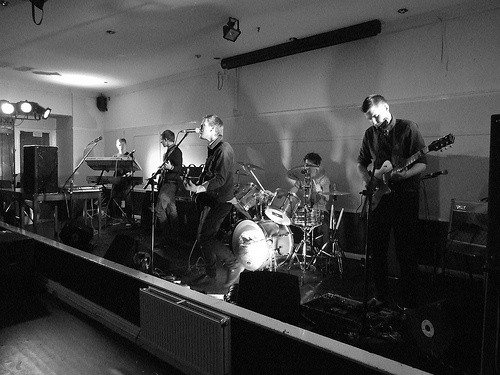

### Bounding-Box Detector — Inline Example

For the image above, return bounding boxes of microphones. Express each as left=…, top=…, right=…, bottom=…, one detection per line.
left=378, top=120, right=388, bottom=129
left=88, top=136, right=102, bottom=145
left=180, top=128, right=200, bottom=134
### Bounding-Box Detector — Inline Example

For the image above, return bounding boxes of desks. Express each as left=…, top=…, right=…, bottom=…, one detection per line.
left=0, top=187, right=102, bottom=238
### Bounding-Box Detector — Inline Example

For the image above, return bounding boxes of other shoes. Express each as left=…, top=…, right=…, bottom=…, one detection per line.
left=191, top=275, right=217, bottom=286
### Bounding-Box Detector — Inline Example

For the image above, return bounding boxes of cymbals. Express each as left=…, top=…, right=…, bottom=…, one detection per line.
left=287, top=164, right=326, bottom=180
left=318, top=190, right=351, bottom=195
left=237, top=162, right=265, bottom=171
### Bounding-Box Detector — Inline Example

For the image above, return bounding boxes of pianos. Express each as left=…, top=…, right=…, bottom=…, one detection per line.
left=84, top=156, right=143, bottom=177
left=85, top=176, right=143, bottom=229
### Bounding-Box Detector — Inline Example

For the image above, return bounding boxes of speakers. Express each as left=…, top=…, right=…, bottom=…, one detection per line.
left=104, top=234, right=138, bottom=265
left=235, top=271, right=300, bottom=321
left=23, top=145, right=58, bottom=195
left=97, top=97, right=107, bottom=112
left=446, top=199, right=488, bottom=257
left=59, top=220, right=94, bottom=249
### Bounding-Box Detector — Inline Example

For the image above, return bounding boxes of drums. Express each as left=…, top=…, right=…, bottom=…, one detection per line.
left=265, top=187, right=301, bottom=225
left=292, top=212, right=323, bottom=227
left=235, top=181, right=265, bottom=220
left=231, top=219, right=294, bottom=272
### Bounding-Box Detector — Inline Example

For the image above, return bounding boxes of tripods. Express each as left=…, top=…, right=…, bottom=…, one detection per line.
left=281, top=177, right=347, bottom=272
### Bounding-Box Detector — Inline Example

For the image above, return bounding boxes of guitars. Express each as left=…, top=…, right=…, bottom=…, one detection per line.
left=183, top=172, right=207, bottom=208
left=157, top=155, right=171, bottom=189
left=359, top=132, right=456, bottom=213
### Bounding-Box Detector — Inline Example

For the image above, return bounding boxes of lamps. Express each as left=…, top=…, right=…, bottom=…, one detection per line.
left=222, top=17, right=242, bottom=42
left=0, top=99, right=51, bottom=124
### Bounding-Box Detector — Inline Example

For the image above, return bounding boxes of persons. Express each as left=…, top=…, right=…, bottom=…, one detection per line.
left=108, top=138, right=136, bottom=226
left=290, top=153, right=331, bottom=245
left=356, top=93, right=426, bottom=313
left=156, top=130, right=182, bottom=249
left=183, top=114, right=245, bottom=288
